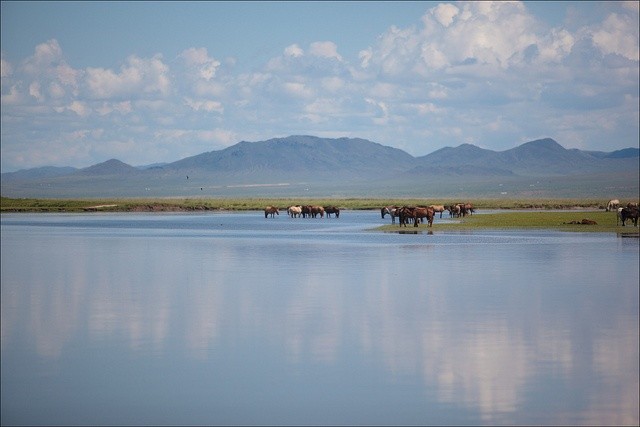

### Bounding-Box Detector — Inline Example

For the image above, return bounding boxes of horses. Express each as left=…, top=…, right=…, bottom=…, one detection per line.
left=443, top=202, right=476, bottom=219
left=264, top=205, right=280, bottom=219
left=616, top=201, right=640, bottom=227
left=287, top=204, right=340, bottom=219
left=605, top=198, right=621, bottom=212
left=380, top=204, right=444, bottom=228
